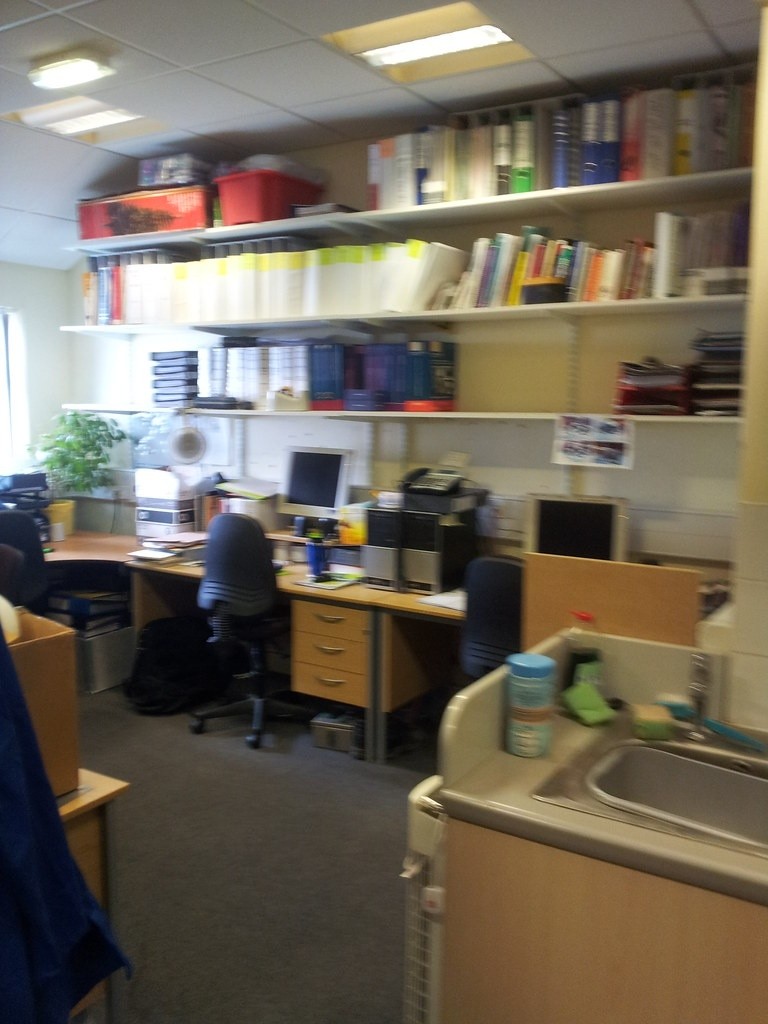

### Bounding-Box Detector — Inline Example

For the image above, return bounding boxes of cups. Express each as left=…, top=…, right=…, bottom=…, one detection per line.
left=291, top=546, right=307, bottom=562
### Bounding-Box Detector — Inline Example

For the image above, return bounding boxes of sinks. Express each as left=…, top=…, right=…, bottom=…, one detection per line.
left=526, top=708, right=768, bottom=860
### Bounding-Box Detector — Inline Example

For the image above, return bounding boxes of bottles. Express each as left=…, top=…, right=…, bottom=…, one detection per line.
left=505, top=653, right=556, bottom=757
left=564, top=612, right=600, bottom=692
left=307, top=534, right=325, bottom=576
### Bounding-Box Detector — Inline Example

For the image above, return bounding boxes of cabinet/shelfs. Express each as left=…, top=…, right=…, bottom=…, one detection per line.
left=59, top=166, right=751, bottom=431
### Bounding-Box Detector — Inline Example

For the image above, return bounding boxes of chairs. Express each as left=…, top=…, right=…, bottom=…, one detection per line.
left=187, top=512, right=316, bottom=749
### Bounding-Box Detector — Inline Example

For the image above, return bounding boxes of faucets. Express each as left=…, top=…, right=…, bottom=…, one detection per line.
left=686, top=652, right=710, bottom=742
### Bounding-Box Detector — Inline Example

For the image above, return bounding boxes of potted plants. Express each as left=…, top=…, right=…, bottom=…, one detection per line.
left=31, top=410, right=127, bottom=533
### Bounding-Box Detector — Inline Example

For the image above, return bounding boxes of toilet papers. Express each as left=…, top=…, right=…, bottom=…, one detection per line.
left=50, top=523, right=64, bottom=542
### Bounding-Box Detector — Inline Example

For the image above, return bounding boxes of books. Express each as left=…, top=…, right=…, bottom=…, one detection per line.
left=127, top=530, right=213, bottom=568
left=83, top=83, right=755, bottom=417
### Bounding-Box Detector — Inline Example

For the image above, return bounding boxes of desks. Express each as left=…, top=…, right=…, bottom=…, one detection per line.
left=54, top=769, right=134, bottom=1022
left=121, top=559, right=472, bottom=766
left=36, top=526, right=137, bottom=564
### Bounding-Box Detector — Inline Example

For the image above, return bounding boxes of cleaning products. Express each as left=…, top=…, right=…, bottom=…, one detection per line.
left=565, top=609, right=602, bottom=710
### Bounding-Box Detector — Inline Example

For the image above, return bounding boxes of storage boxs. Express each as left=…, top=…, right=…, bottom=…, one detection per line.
left=4, top=610, right=80, bottom=800
left=76, top=185, right=214, bottom=241
left=210, top=170, right=323, bottom=226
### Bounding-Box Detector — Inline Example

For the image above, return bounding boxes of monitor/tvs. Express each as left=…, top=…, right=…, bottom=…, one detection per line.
left=278, top=446, right=353, bottom=540
left=525, top=493, right=629, bottom=563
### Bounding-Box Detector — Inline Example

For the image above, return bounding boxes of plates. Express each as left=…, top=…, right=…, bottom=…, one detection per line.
left=127, top=550, right=175, bottom=560
